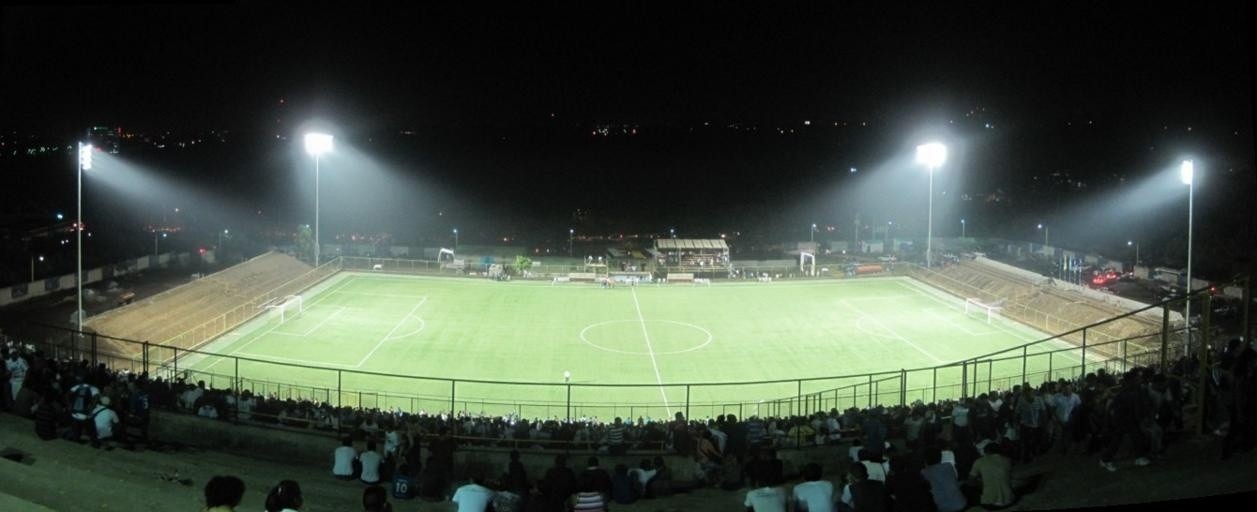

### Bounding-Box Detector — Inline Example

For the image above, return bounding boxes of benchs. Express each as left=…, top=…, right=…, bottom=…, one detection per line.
left=0, top=383, right=761, bottom=510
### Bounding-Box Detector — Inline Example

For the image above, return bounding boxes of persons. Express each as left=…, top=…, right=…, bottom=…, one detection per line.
left=468, top=249, right=960, bottom=289
left=0, top=337, right=1257, bottom=512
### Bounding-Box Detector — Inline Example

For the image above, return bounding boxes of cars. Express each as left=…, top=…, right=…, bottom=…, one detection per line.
left=1152, top=282, right=1242, bottom=321
left=876, top=253, right=897, bottom=261
left=1059, top=258, right=1123, bottom=286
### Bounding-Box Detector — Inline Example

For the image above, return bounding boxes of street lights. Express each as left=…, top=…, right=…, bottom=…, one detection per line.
left=1176, top=156, right=1198, bottom=356
left=302, top=130, right=334, bottom=267
left=914, top=139, right=949, bottom=271
left=73, top=140, right=94, bottom=340
left=1126, top=239, right=1139, bottom=264
left=1036, top=223, right=1048, bottom=245
left=218, top=229, right=229, bottom=253
left=669, top=226, right=676, bottom=239
left=452, top=228, right=458, bottom=248
left=568, top=228, right=574, bottom=255
left=959, top=217, right=965, bottom=237
left=809, top=223, right=815, bottom=240
